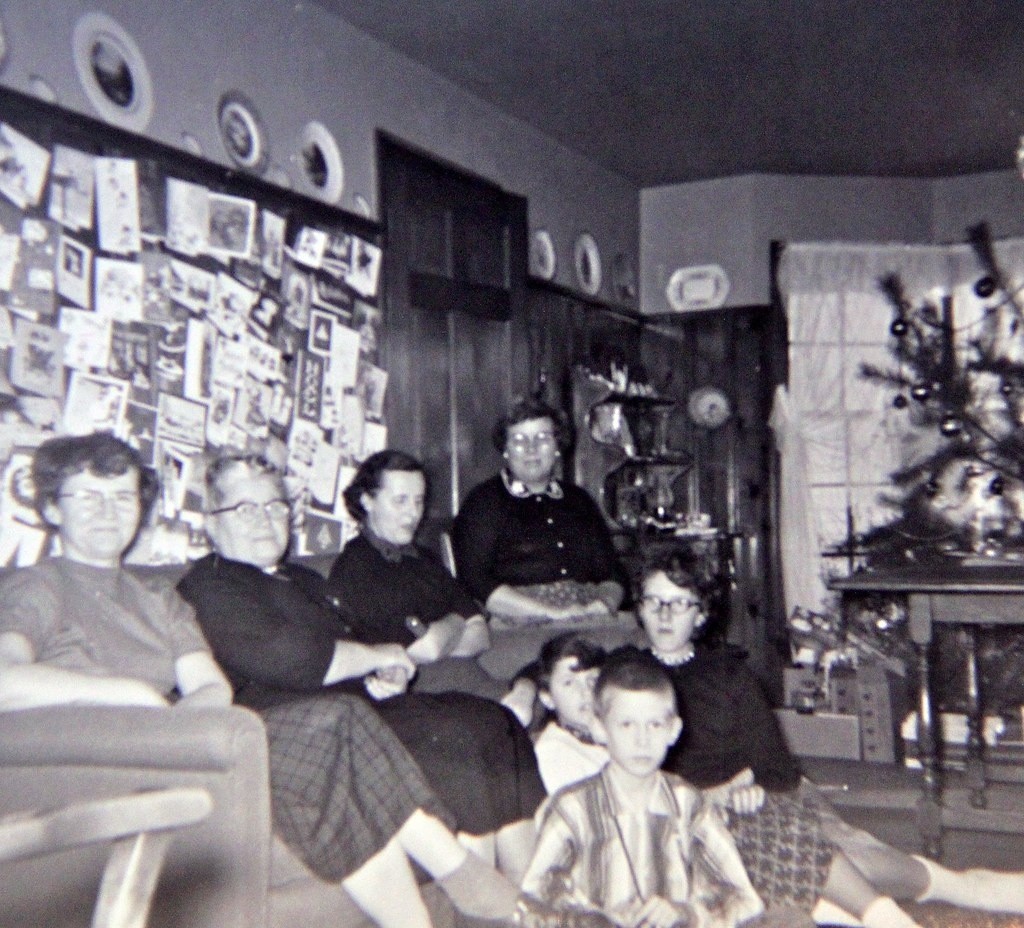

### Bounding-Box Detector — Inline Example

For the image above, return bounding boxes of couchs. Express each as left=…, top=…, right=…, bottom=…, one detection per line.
left=1, top=547, right=652, bottom=928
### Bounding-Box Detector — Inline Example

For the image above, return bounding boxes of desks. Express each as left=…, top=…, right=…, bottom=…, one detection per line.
left=828, top=569, right=1024, bottom=862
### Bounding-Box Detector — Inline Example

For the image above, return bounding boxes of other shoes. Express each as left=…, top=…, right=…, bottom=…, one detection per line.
left=859, top=894, right=920, bottom=928
left=913, top=851, right=1024, bottom=913
left=438, top=847, right=517, bottom=923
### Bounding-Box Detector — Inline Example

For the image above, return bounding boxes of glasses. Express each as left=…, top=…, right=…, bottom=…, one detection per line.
left=507, top=430, right=556, bottom=448
left=605, top=711, right=669, bottom=736
left=209, top=498, right=292, bottom=522
left=56, top=487, right=141, bottom=507
left=638, top=594, right=704, bottom=614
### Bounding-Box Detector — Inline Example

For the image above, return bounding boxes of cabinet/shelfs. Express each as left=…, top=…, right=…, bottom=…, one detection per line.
left=569, top=366, right=725, bottom=592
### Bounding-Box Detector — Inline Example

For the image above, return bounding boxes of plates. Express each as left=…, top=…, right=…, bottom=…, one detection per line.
left=272, top=165, right=292, bottom=192
left=299, top=119, right=346, bottom=203
left=213, top=87, right=271, bottom=172
left=72, top=12, right=154, bottom=132
left=180, top=130, right=202, bottom=158
left=574, top=229, right=604, bottom=295
left=351, top=191, right=373, bottom=219
left=610, top=253, right=639, bottom=303
left=0, top=17, right=15, bottom=82
left=28, top=72, right=57, bottom=104
left=665, top=264, right=731, bottom=311
left=531, top=227, right=556, bottom=281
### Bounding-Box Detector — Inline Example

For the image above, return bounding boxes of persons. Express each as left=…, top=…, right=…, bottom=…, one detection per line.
left=495, top=550, right=1021, bottom=928
left=455, top=401, right=634, bottom=671
left=533, top=631, right=612, bottom=797
left=526, top=661, right=768, bottom=928
left=325, top=452, right=542, bottom=743
left=0, top=428, right=523, bottom=927
left=178, top=456, right=546, bottom=879
